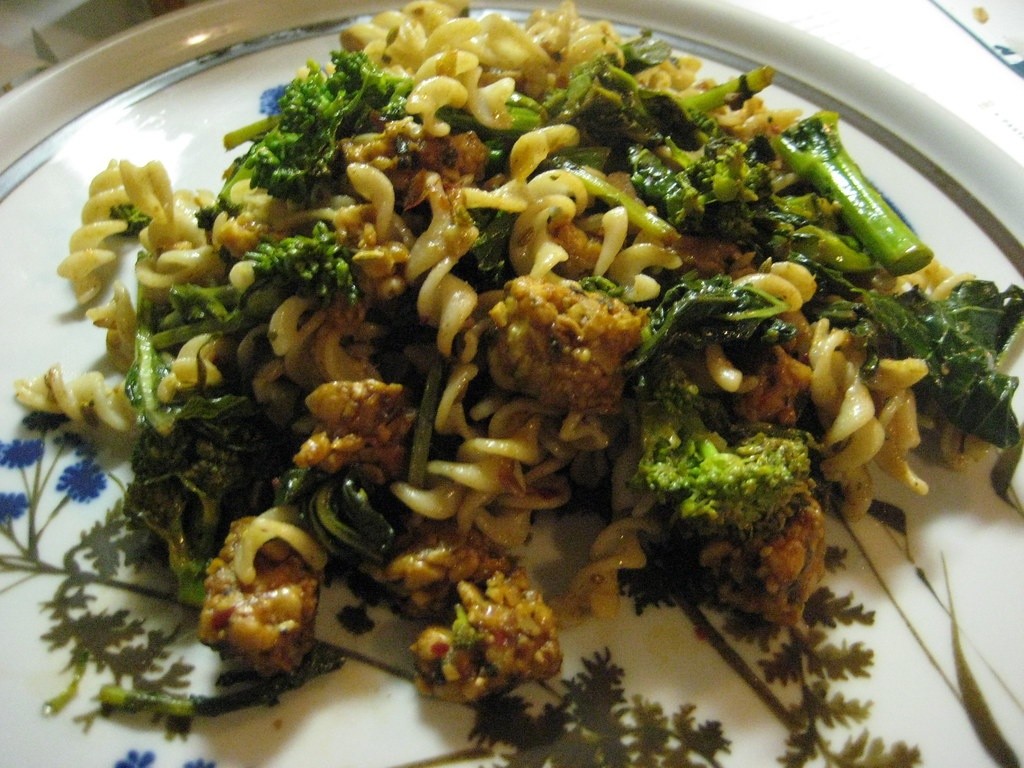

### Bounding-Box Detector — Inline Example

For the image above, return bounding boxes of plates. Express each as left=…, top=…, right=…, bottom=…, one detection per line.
left=0, top=0, right=1024, bottom=768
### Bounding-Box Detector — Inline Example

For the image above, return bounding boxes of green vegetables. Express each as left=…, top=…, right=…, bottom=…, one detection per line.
left=41, top=50, right=1024, bottom=717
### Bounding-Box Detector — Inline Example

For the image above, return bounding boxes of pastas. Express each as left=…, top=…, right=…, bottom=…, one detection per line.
left=9, top=0, right=931, bottom=527
